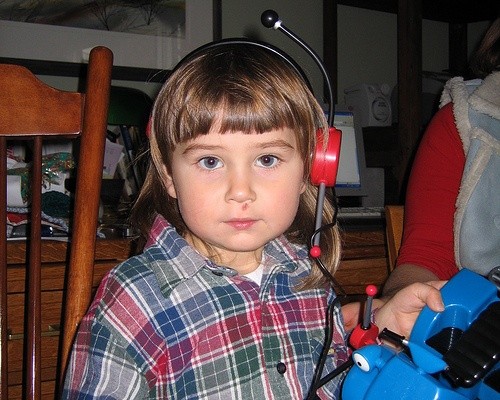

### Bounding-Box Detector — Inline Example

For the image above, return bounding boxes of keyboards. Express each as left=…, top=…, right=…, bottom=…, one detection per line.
left=336, top=206, right=385, bottom=218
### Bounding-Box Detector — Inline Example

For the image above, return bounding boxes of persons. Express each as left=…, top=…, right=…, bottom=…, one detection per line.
left=340, top=17, right=500, bottom=333
left=63, top=43, right=446, bottom=399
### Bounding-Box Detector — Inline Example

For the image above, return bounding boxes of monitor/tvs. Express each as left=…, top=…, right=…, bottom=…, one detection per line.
left=324, top=111, right=370, bottom=197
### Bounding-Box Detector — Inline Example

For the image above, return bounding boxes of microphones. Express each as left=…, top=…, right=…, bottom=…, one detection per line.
left=261, top=10, right=342, bottom=257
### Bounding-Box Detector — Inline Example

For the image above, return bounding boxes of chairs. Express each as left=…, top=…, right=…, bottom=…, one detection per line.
left=0, top=45, right=114, bottom=400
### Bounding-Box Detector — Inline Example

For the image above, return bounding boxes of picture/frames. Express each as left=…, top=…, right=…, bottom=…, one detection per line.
left=0, top=0, right=222, bottom=84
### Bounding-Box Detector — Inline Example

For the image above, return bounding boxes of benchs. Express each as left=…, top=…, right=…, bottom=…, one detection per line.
left=0, top=205, right=405, bottom=400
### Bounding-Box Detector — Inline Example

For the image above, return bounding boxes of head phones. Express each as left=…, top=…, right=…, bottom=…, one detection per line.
left=145, top=39, right=343, bottom=187
